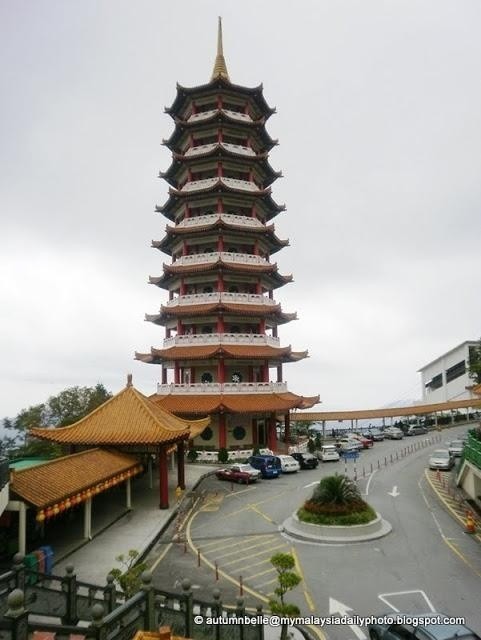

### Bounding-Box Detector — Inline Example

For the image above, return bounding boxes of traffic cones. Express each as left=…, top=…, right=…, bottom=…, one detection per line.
left=465, top=509, right=477, bottom=533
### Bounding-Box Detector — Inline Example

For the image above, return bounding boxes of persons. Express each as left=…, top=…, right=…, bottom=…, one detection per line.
left=176, top=485, right=182, bottom=505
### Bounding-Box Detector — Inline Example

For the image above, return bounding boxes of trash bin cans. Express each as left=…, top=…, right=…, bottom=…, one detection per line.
left=24, top=545, right=53, bottom=585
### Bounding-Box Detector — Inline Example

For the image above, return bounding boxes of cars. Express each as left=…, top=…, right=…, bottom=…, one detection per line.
left=430, top=427, right=466, bottom=470
left=216, top=421, right=426, bottom=485
left=366, top=610, right=481, bottom=639
